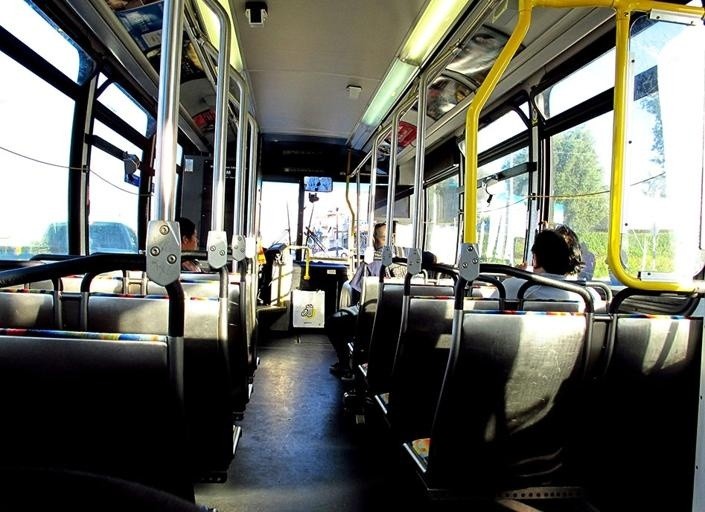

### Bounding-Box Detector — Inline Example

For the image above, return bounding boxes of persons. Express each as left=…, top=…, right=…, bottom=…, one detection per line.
left=490, top=224, right=602, bottom=300
left=324, top=223, right=397, bottom=378
left=605, top=250, right=628, bottom=285
left=577, top=241, right=596, bottom=281
left=175, top=217, right=202, bottom=272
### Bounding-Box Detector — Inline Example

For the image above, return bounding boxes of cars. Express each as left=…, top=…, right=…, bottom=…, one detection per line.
left=314, top=245, right=354, bottom=260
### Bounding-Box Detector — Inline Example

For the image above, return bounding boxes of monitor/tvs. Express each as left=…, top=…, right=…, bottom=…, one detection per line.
left=303, top=175, right=333, bottom=192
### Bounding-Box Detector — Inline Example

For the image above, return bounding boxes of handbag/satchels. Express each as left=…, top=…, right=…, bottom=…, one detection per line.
left=292, top=289, right=325, bottom=328
